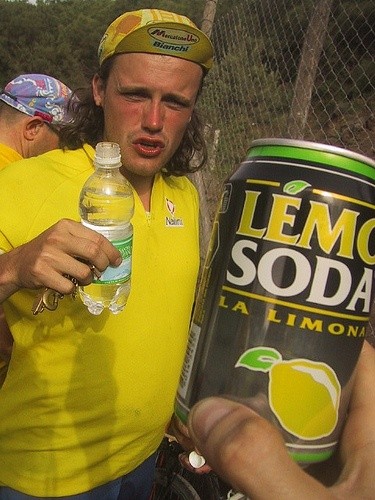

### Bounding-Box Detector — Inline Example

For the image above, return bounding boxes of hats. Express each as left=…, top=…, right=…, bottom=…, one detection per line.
left=0, top=74, right=86, bottom=142
left=98, top=8, right=214, bottom=72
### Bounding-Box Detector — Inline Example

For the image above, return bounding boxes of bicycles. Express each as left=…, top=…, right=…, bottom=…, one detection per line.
left=146, top=433, right=229, bottom=500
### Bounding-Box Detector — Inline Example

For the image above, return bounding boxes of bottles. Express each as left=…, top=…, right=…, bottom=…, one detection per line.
left=77, top=143, right=134, bottom=316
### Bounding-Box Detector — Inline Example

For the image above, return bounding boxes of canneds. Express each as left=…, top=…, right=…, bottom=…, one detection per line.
left=172, top=137, right=375, bottom=464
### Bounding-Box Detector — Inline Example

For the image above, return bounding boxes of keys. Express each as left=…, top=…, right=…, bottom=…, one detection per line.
left=32, top=265, right=101, bottom=315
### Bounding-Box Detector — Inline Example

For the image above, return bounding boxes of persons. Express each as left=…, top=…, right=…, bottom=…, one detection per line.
left=0, top=74, right=80, bottom=387
left=0, top=8, right=216, bottom=500
left=186, top=338, right=375, bottom=500
